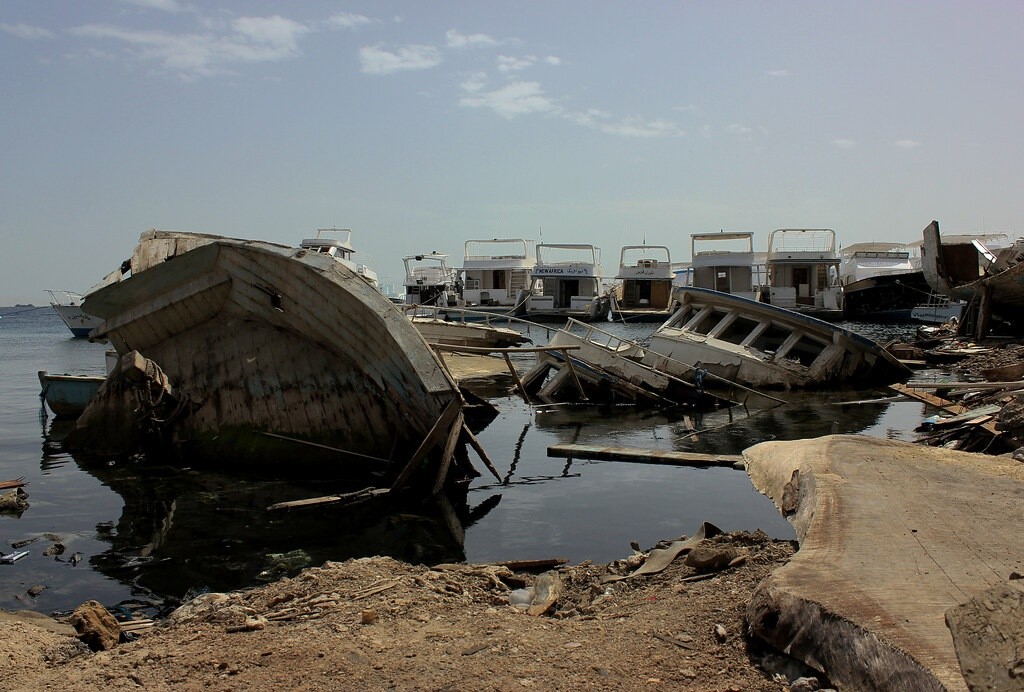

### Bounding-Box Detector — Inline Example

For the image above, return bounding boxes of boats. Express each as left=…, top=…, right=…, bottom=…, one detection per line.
left=508, top=315, right=788, bottom=417
left=609, top=245, right=675, bottom=318
left=765, top=226, right=846, bottom=313
left=43, top=289, right=106, bottom=338
left=36, top=369, right=108, bottom=417
left=69, top=229, right=501, bottom=498
left=837, top=249, right=931, bottom=317
left=645, top=286, right=915, bottom=389
left=681, top=229, right=762, bottom=303
left=401, top=252, right=465, bottom=317
left=299, top=228, right=379, bottom=287
left=458, top=239, right=542, bottom=310
left=949, top=242, right=1024, bottom=339
left=515, top=243, right=609, bottom=315
left=407, top=316, right=534, bottom=357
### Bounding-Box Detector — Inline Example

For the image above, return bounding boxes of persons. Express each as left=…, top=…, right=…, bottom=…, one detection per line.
left=455, top=277, right=464, bottom=296
